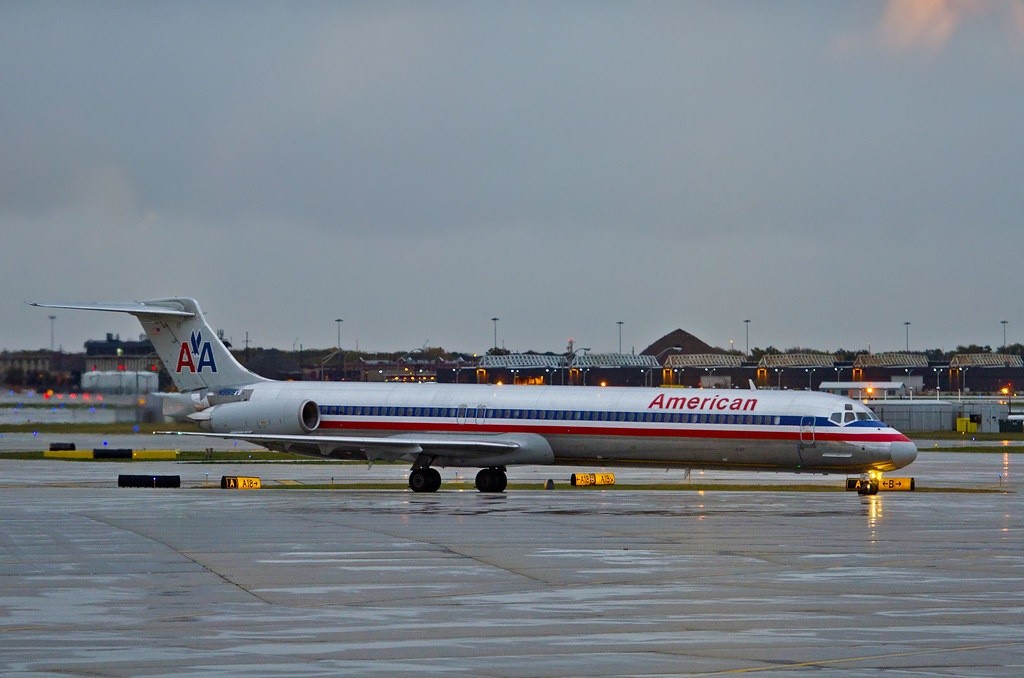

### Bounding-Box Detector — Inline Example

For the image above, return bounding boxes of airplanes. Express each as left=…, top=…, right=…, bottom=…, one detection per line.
left=25, top=296, right=919, bottom=496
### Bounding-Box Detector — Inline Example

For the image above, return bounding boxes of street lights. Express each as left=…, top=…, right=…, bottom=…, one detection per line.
left=744, top=319, right=751, bottom=357
left=491, top=317, right=499, bottom=347
left=904, top=321, right=911, bottom=353
left=336, top=318, right=343, bottom=348
left=617, top=321, right=625, bottom=354
left=1001, top=320, right=1009, bottom=348
